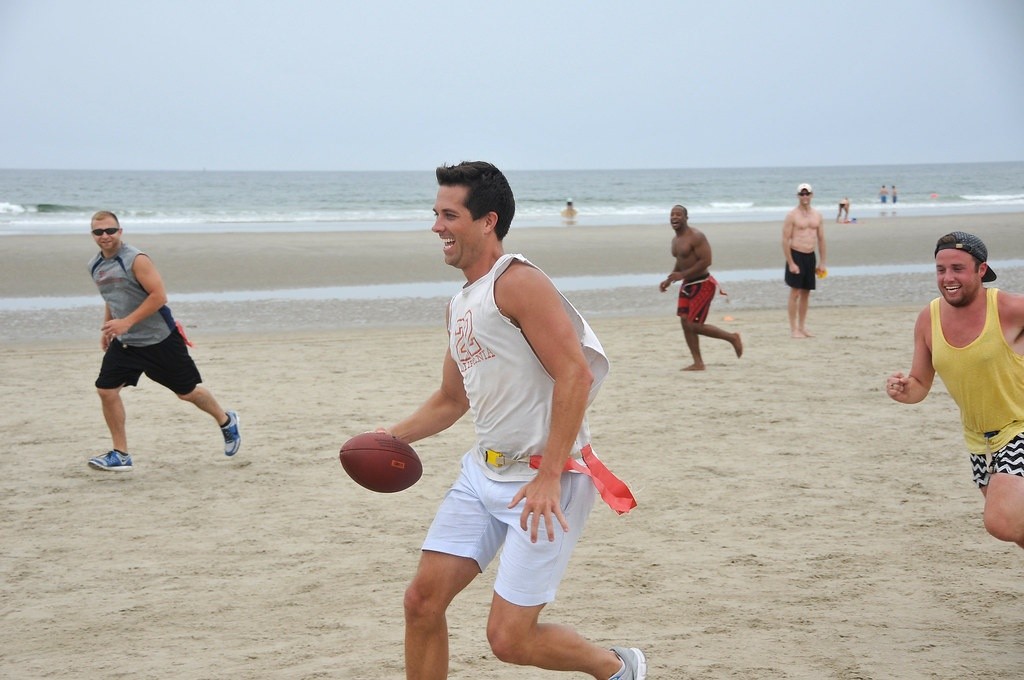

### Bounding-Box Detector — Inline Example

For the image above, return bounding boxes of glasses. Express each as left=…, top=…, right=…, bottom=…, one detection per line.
left=91, top=227, right=119, bottom=236
left=798, top=192, right=809, bottom=196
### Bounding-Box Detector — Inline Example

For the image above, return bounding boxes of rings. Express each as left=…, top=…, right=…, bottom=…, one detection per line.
left=889, top=384, right=893, bottom=388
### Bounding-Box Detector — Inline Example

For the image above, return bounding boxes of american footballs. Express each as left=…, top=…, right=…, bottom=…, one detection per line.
left=338, top=432, right=425, bottom=494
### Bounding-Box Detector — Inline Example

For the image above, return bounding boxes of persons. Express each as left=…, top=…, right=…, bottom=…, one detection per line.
left=781, top=183, right=827, bottom=339
left=888, top=232, right=1024, bottom=549
left=878, top=185, right=888, bottom=203
left=891, top=186, right=897, bottom=203
left=659, top=205, right=743, bottom=371
left=367, top=162, right=646, bottom=680
left=86, top=212, right=240, bottom=472
left=560, top=198, right=576, bottom=215
left=835, top=197, right=850, bottom=224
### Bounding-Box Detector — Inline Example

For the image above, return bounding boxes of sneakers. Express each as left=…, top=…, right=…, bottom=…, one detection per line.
left=220, top=411, right=240, bottom=457
left=88, top=450, right=133, bottom=472
left=608, top=647, right=646, bottom=680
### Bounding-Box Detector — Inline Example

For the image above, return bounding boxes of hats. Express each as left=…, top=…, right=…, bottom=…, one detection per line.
left=934, top=231, right=997, bottom=283
left=797, top=183, right=812, bottom=194
